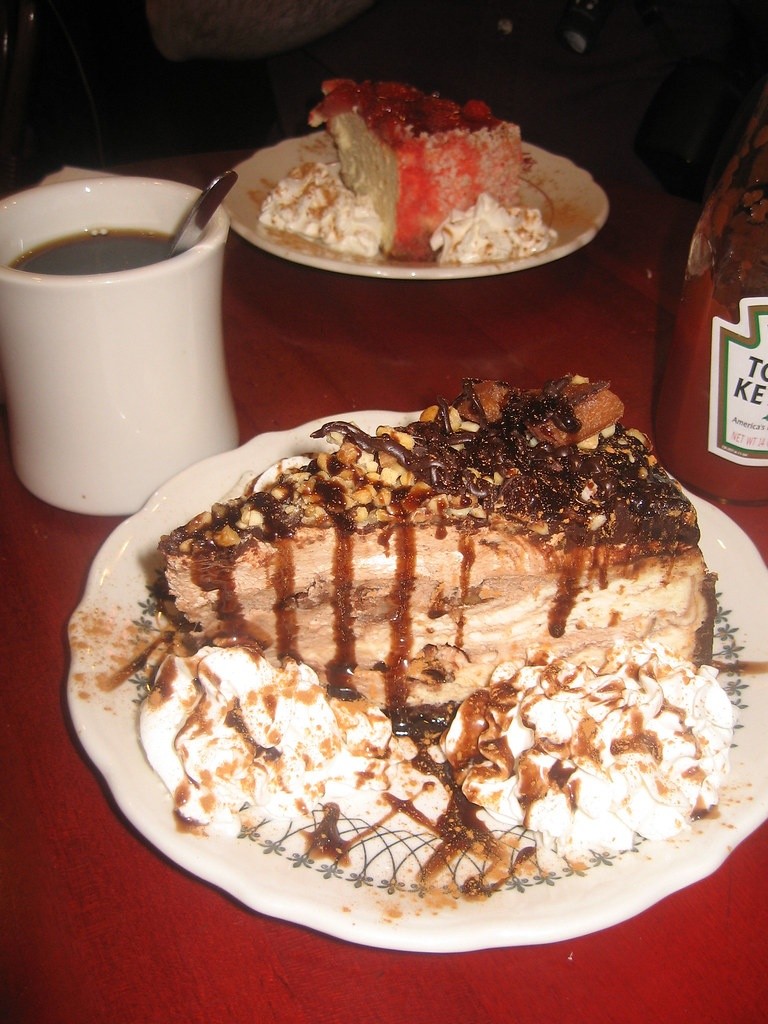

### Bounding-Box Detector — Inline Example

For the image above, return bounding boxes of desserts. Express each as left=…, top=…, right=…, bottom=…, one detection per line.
left=253, top=77, right=561, bottom=265
left=136, top=371, right=736, bottom=865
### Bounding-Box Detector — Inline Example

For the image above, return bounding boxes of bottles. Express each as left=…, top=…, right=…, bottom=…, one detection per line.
left=653, top=85, right=768, bottom=504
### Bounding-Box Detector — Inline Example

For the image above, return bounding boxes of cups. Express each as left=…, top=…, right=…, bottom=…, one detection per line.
left=0, top=165, right=258, bottom=515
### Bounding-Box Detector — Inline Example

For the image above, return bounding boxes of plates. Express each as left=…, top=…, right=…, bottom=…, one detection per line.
left=223, top=127, right=609, bottom=279
left=65, top=409, right=768, bottom=955
